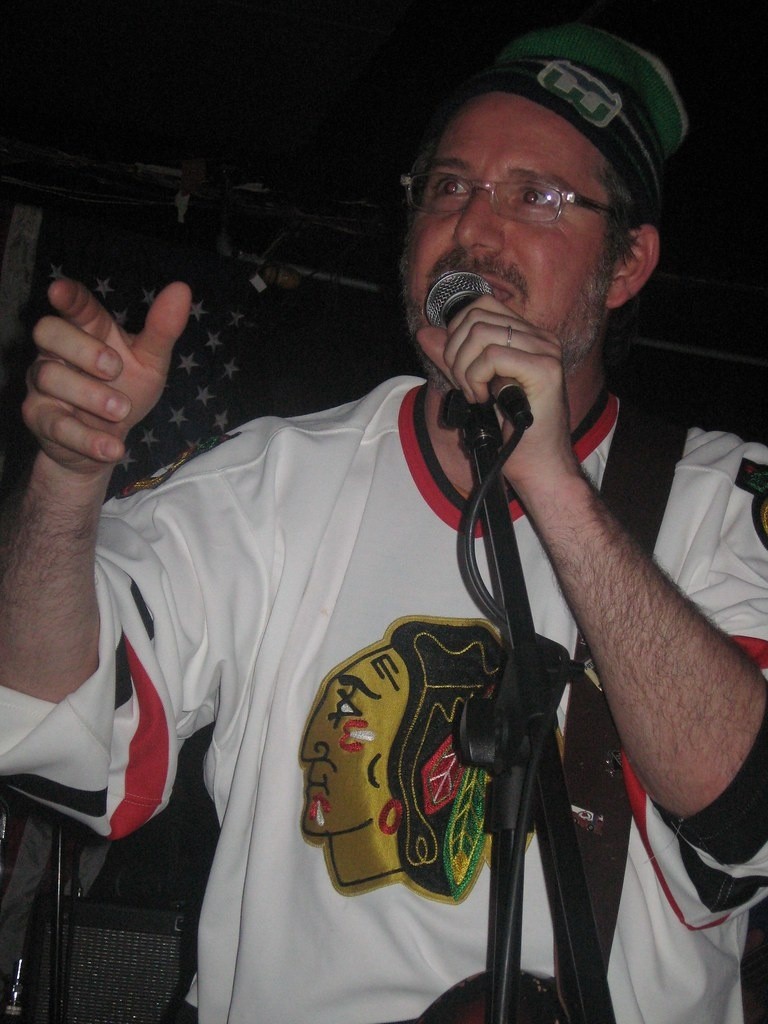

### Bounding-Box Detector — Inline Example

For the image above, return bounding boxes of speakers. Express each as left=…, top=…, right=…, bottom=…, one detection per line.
left=30, top=897, right=196, bottom=1023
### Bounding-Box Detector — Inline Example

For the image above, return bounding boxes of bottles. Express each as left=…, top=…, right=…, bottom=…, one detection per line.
left=2, top=955, right=31, bottom=1024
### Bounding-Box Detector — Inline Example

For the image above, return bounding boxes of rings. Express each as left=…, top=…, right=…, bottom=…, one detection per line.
left=507, top=326, right=512, bottom=348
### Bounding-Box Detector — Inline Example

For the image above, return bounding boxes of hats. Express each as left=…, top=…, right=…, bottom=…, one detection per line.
left=441, top=20, right=690, bottom=226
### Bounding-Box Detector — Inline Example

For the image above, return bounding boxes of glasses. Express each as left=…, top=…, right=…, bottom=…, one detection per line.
left=398, top=169, right=623, bottom=226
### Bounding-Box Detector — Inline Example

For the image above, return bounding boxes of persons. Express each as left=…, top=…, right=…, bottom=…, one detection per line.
left=1, top=30, right=768, bottom=1021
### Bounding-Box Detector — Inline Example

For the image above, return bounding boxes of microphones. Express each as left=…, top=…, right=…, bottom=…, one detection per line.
left=426, top=271, right=534, bottom=431
